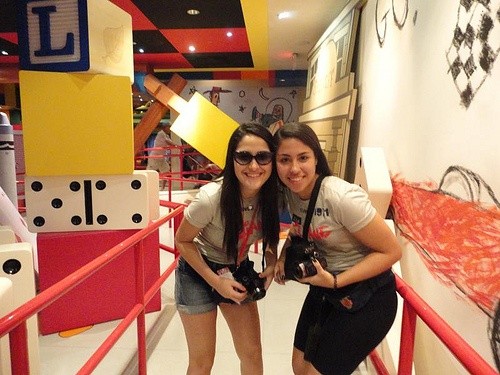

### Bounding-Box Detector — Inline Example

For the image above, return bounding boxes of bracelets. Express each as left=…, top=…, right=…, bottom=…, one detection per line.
left=332, top=273, right=337, bottom=291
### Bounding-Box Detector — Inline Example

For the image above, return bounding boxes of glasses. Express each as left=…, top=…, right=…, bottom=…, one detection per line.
left=234, top=151, right=273, bottom=165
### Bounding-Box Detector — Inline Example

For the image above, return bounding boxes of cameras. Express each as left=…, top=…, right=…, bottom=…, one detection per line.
left=232, top=258, right=266, bottom=301
left=292, top=241, right=328, bottom=279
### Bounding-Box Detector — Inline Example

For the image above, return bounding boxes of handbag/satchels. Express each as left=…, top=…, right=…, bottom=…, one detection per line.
left=283, top=235, right=310, bottom=280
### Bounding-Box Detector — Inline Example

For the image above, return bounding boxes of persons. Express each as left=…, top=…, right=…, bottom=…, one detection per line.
left=170, top=123, right=281, bottom=375
left=146, top=120, right=177, bottom=177
left=271, top=121, right=404, bottom=375
